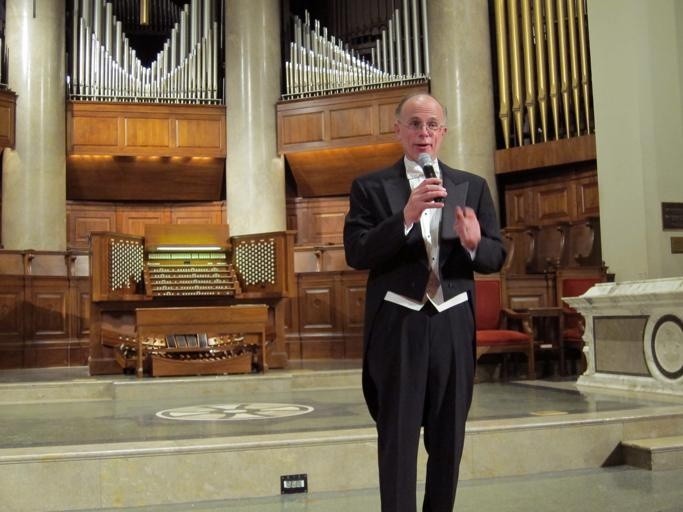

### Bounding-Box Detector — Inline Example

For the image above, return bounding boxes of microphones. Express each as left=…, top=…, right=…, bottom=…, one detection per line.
left=418, top=152, right=445, bottom=202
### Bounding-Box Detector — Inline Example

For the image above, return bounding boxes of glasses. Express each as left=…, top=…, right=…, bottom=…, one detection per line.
left=397, top=119, right=444, bottom=132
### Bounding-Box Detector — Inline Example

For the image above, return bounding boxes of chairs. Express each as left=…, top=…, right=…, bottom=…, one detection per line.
left=474, top=269, right=613, bottom=379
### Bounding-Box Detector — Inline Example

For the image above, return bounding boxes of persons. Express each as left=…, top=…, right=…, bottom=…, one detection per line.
left=343, top=94, right=507, bottom=511
